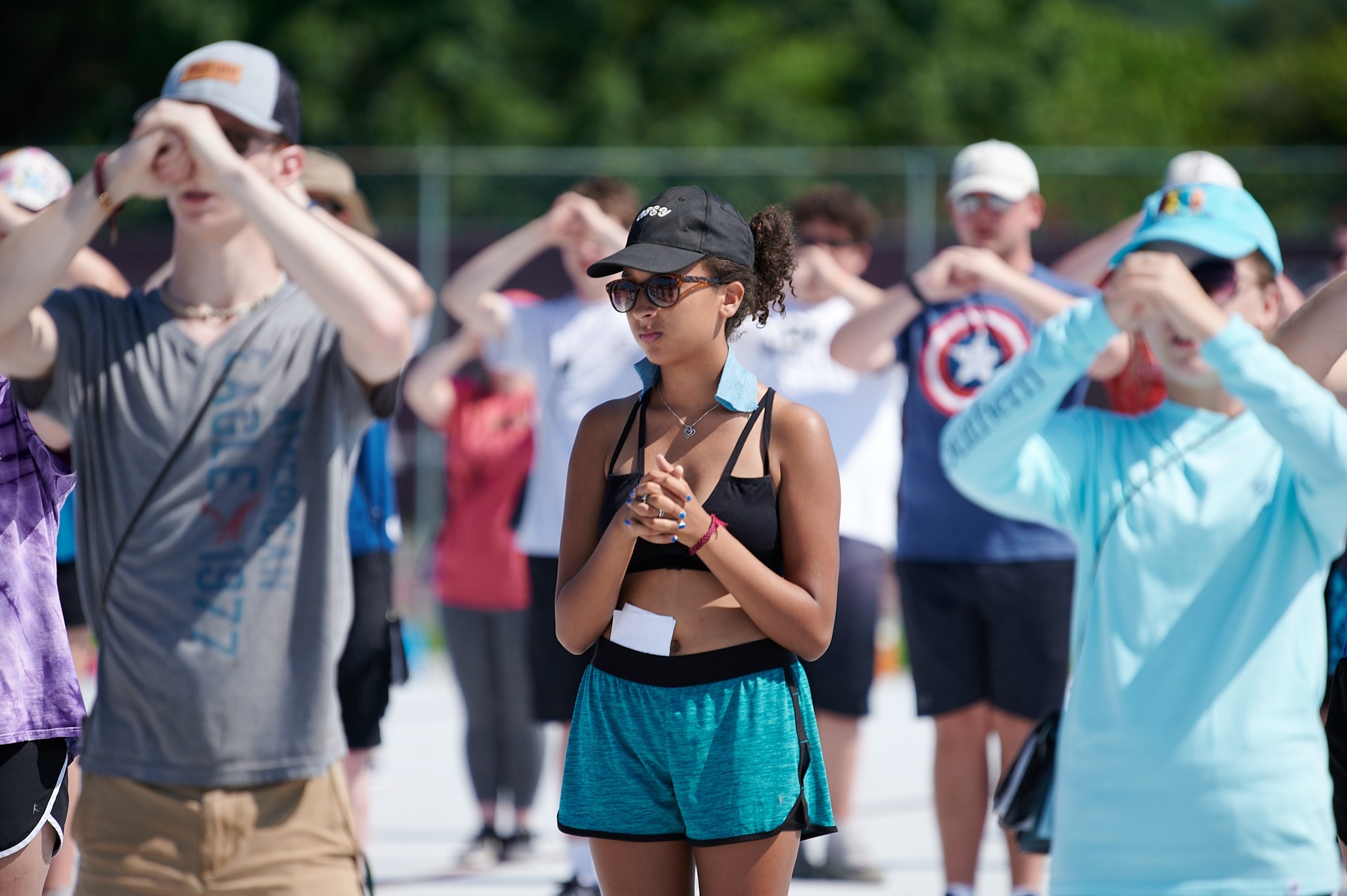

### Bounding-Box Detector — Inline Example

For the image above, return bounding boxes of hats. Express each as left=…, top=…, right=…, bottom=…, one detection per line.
left=948, top=139, right=1040, bottom=202
left=136, top=40, right=303, bottom=145
left=586, top=186, right=754, bottom=278
left=1168, top=151, right=1243, bottom=185
left=0, top=147, right=72, bottom=210
left=300, top=149, right=354, bottom=195
left=1109, top=183, right=1283, bottom=276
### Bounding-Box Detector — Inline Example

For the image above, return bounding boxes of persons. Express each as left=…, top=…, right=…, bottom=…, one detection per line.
left=1, top=38, right=1347, bottom=896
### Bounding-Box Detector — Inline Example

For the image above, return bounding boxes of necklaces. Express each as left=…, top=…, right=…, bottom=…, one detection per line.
left=655, top=381, right=721, bottom=439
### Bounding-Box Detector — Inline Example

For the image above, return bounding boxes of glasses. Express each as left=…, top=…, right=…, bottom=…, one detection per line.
left=225, top=131, right=279, bottom=154
left=956, top=194, right=1011, bottom=215
left=800, top=236, right=857, bottom=250
left=606, top=272, right=728, bottom=313
left=1186, top=256, right=1264, bottom=305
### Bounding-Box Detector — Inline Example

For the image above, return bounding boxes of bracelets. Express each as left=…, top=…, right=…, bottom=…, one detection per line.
left=902, top=273, right=936, bottom=310
left=93, top=153, right=125, bottom=213
left=687, top=512, right=728, bottom=555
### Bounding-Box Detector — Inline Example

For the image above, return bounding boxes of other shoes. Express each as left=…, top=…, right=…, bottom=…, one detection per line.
left=463, top=824, right=540, bottom=869
left=792, top=829, right=883, bottom=883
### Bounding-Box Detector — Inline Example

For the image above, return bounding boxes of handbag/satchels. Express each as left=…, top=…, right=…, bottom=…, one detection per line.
left=992, top=713, right=1062, bottom=832
left=390, top=616, right=409, bottom=684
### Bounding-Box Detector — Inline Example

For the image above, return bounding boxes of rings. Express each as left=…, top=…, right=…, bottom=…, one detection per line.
left=657, top=508, right=664, bottom=518
left=642, top=493, right=649, bottom=503
